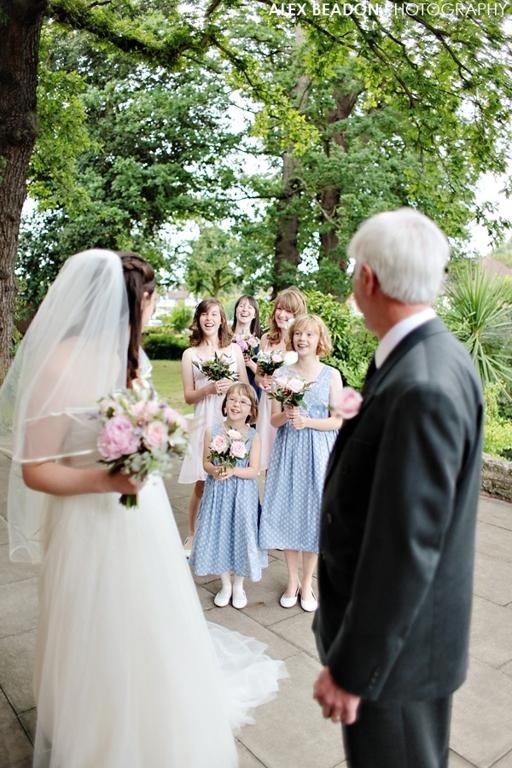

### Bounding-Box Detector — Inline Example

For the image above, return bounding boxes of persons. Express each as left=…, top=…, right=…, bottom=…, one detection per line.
left=0, top=248, right=292, bottom=768
left=254, top=288, right=308, bottom=553
left=257, top=313, right=344, bottom=612
left=189, top=383, right=270, bottom=610
left=179, top=298, right=251, bottom=559
left=228, top=294, right=262, bottom=431
left=309, top=206, right=486, bottom=768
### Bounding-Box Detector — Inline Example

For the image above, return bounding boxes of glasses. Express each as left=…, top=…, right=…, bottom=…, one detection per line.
left=229, top=395, right=253, bottom=407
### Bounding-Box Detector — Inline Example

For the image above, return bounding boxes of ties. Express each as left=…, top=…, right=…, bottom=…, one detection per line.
left=365, top=354, right=376, bottom=388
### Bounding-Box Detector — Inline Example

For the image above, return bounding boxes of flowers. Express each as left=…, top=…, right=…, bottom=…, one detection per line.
left=235, top=333, right=259, bottom=353
left=334, top=387, right=363, bottom=418
left=96, top=378, right=187, bottom=506
left=272, top=369, right=316, bottom=408
left=257, top=351, right=284, bottom=375
left=209, top=429, right=247, bottom=466
left=201, top=350, right=234, bottom=381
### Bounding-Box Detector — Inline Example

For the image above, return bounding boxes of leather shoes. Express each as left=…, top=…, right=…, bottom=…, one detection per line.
left=232, top=588, right=247, bottom=608
left=299, top=587, right=318, bottom=611
left=214, top=587, right=233, bottom=607
left=182, top=537, right=194, bottom=559
left=280, top=581, right=301, bottom=608
